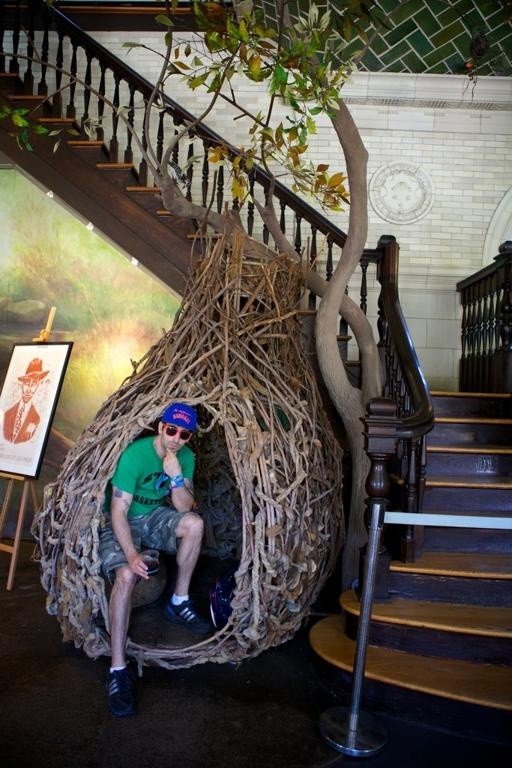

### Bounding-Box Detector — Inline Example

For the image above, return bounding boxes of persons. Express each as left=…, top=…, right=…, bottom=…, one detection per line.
left=2, top=359, right=49, bottom=443
left=99, top=403, right=212, bottom=716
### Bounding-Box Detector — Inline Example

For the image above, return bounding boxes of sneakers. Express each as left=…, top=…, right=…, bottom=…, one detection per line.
left=165, top=599, right=211, bottom=635
left=105, top=665, right=136, bottom=718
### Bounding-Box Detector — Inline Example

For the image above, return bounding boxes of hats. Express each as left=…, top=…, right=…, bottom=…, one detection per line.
left=160, top=402, right=199, bottom=433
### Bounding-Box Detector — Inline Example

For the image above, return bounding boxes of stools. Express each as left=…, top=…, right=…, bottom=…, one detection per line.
left=101, top=548, right=168, bottom=608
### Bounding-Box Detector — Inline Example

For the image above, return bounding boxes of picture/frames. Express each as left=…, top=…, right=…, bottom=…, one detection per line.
left=0, top=341, right=73, bottom=480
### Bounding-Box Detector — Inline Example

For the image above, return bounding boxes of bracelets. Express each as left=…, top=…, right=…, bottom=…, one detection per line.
left=168, top=474, right=185, bottom=488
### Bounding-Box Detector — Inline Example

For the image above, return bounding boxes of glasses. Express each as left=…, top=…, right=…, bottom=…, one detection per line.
left=161, top=426, right=192, bottom=442
